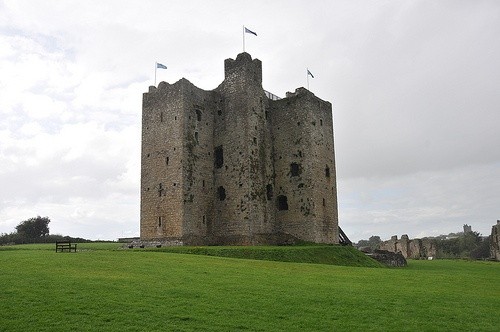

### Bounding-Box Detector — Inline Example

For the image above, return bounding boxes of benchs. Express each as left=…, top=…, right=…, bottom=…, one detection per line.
left=55, top=240, right=77, bottom=254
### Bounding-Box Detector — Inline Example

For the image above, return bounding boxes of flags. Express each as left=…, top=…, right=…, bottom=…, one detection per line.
left=245, top=27, right=257, bottom=37
left=307, top=68, right=314, bottom=79
left=156, top=63, right=168, bottom=70
